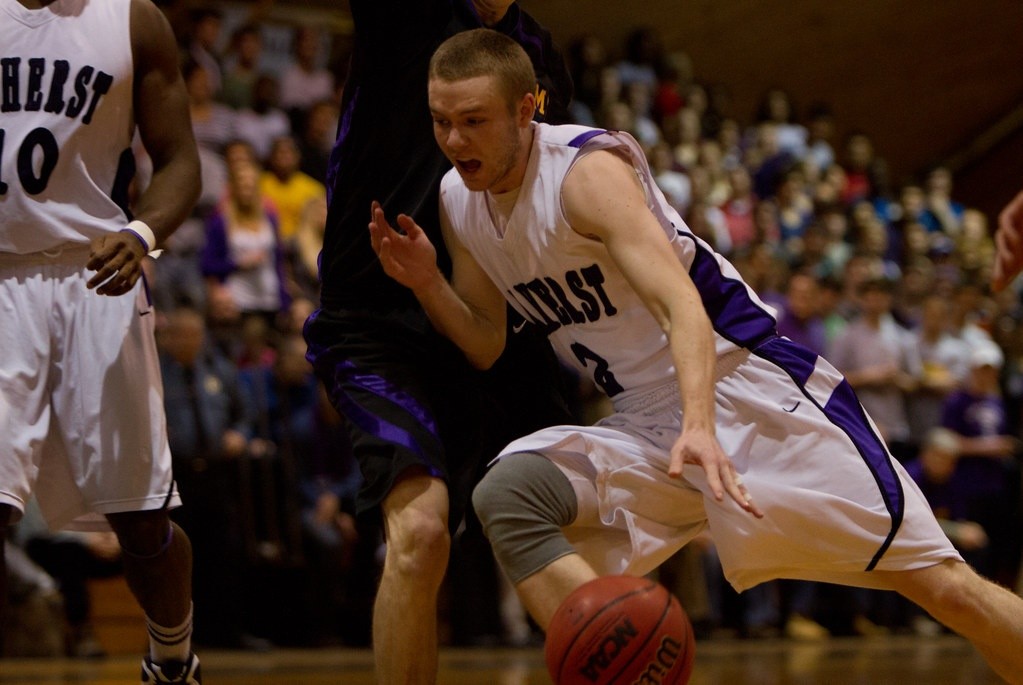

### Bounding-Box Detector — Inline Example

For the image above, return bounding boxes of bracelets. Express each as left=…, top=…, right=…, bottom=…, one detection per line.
left=121, top=219, right=163, bottom=260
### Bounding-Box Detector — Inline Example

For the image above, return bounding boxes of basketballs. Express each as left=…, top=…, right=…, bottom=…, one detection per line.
left=545, top=577, right=697, bottom=685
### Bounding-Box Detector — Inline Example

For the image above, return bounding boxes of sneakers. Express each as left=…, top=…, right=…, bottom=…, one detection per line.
left=141, top=651, right=203, bottom=685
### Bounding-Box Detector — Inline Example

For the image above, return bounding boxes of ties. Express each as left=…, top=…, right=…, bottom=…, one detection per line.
left=186, top=366, right=213, bottom=458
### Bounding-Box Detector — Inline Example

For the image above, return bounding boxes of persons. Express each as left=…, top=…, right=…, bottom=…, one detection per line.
left=0, top=0, right=1023, bottom=685
